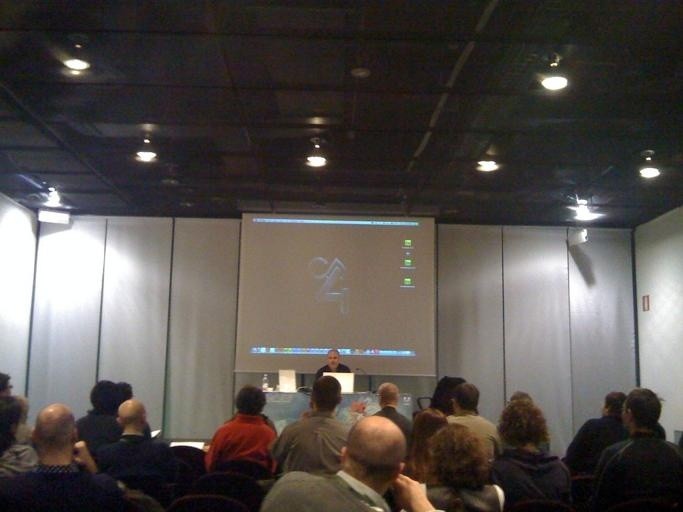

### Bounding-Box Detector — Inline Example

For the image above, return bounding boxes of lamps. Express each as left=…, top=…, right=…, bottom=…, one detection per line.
left=638, top=149, right=662, bottom=179
left=62, top=32, right=93, bottom=72
left=135, top=133, right=157, bottom=162
left=305, top=137, right=328, bottom=169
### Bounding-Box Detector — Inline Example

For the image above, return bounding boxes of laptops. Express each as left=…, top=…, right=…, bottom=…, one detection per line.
left=323, top=371, right=355, bottom=394
left=279, top=368, right=298, bottom=393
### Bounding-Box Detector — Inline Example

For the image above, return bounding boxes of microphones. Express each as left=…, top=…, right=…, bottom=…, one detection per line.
left=356, top=367, right=372, bottom=392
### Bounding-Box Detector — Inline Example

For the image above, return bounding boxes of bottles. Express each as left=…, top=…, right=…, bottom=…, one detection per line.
left=261, top=374, right=268, bottom=391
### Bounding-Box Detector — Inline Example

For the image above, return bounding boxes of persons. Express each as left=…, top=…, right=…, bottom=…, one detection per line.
left=206, top=376, right=572, bottom=512
left=316, top=348, right=351, bottom=381
left=565, top=388, right=683, bottom=512
left=0, top=373, right=177, bottom=512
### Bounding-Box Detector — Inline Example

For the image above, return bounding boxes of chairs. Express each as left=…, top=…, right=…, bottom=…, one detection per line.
left=507, top=498, right=578, bottom=512
left=173, top=494, right=249, bottom=511
left=173, top=446, right=204, bottom=465
left=215, top=458, right=273, bottom=477
left=193, top=471, right=265, bottom=495
